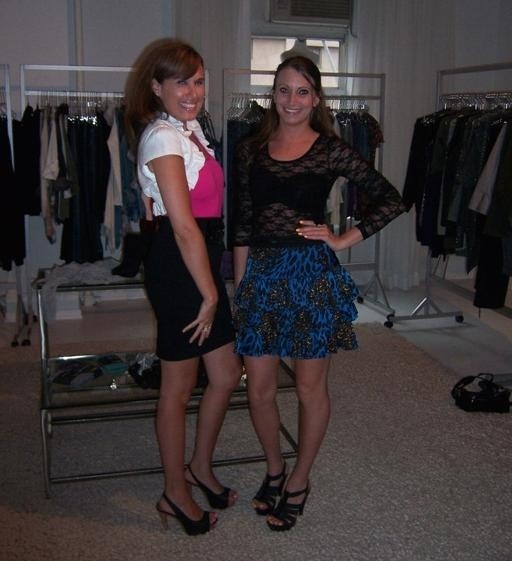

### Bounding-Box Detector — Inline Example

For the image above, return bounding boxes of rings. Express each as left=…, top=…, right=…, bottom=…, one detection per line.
left=202, top=326, right=209, bottom=331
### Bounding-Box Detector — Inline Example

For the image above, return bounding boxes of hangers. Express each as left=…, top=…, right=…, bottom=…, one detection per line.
left=1, top=87, right=126, bottom=116
left=430, top=92, right=511, bottom=119
left=227, top=92, right=371, bottom=122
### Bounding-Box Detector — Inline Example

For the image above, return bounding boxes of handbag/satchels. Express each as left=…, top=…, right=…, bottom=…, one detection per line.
left=449, top=371, right=511, bottom=415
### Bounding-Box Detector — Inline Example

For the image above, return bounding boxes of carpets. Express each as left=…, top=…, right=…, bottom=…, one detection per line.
left=1, top=322, right=512, bottom=561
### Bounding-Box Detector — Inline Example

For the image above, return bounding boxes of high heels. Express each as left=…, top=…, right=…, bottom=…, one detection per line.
left=184, top=463, right=239, bottom=510
left=155, top=488, right=219, bottom=536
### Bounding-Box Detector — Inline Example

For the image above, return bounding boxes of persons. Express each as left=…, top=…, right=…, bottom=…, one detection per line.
left=123, top=39, right=239, bottom=535
left=231, top=55, right=404, bottom=532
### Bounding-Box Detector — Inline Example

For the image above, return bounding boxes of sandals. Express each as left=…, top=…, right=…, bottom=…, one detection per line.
left=266, top=477, right=312, bottom=532
left=250, top=457, right=290, bottom=516
